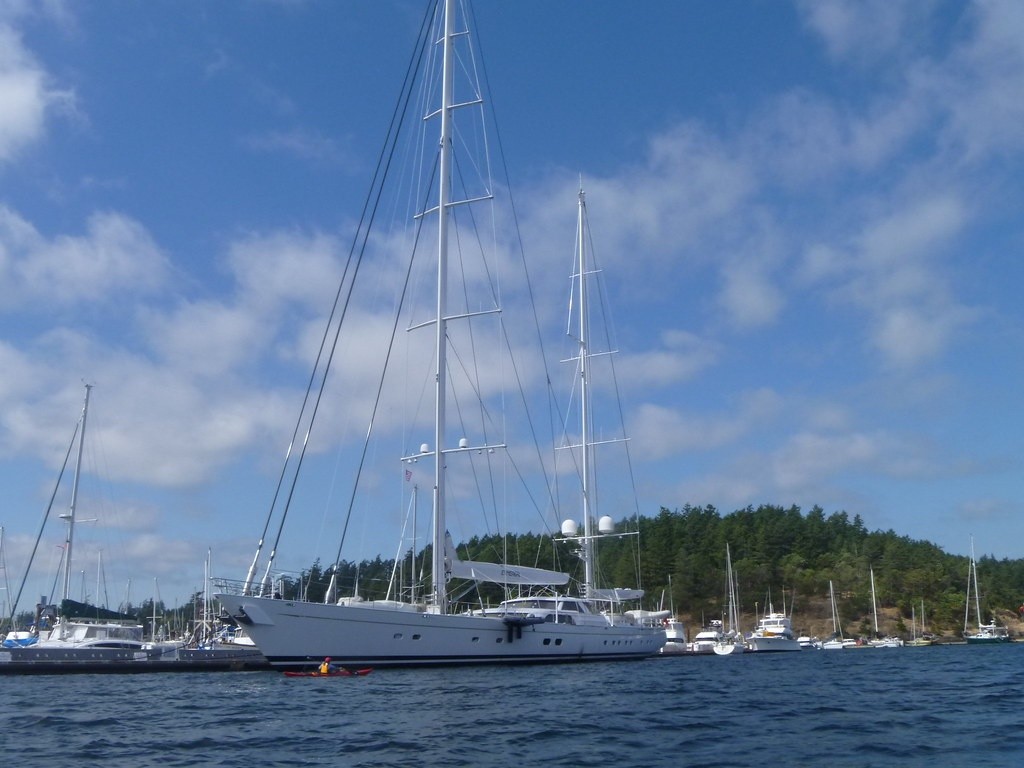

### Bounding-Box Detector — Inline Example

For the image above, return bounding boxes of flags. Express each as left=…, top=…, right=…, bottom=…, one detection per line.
left=405, top=469, right=413, bottom=483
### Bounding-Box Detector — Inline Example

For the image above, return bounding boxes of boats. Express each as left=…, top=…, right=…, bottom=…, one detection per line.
left=283, top=666, right=375, bottom=678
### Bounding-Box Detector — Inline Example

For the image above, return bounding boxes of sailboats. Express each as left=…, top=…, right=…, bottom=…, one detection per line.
left=645, top=536, right=1012, bottom=657
left=210, top=0, right=668, bottom=671
left=1, top=381, right=280, bottom=650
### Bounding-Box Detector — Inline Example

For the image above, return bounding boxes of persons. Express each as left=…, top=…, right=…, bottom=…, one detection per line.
left=318, top=656, right=344, bottom=676
